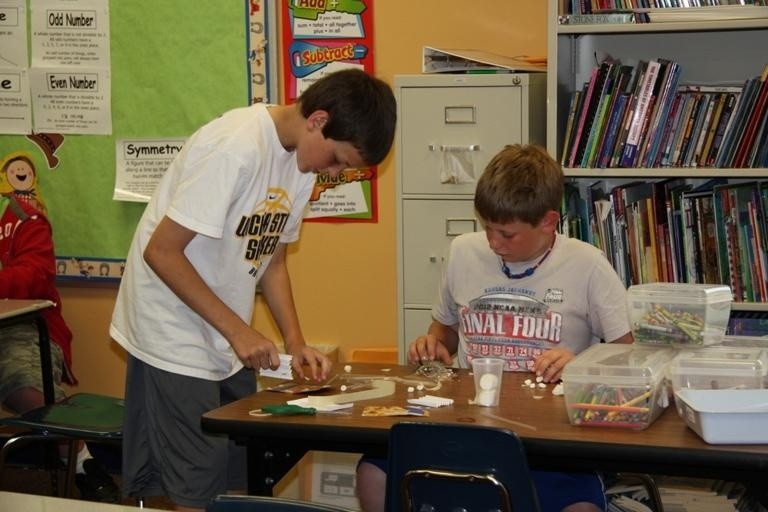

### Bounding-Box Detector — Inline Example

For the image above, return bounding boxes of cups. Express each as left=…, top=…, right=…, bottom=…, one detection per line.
left=470, top=357, right=504, bottom=409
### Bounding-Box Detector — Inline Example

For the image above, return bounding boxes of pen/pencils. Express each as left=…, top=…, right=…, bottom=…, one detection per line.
left=676, top=379, right=754, bottom=391
left=633, top=303, right=725, bottom=346
left=568, top=381, right=669, bottom=430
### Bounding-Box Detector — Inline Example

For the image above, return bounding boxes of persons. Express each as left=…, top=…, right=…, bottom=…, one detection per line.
left=108, top=66, right=397, bottom=510
left=354, top=142, right=636, bottom=512
left=0, top=192, right=121, bottom=503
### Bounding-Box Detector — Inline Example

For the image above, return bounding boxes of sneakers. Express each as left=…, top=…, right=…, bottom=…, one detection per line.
left=74, top=458, right=121, bottom=504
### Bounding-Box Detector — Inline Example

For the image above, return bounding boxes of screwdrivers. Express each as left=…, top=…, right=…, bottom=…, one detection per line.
left=262, top=405, right=352, bottom=416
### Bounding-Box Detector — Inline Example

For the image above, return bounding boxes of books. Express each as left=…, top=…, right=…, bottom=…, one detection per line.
left=605, top=471, right=760, bottom=512
left=557, top=177, right=768, bottom=303
left=560, top=50, right=768, bottom=168
left=557, top=1, right=768, bottom=25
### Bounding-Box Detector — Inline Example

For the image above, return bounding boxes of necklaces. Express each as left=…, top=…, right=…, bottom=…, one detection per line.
left=501, top=228, right=556, bottom=279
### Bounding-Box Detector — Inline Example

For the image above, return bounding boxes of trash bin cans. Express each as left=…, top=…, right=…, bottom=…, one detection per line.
left=346, top=348, right=398, bottom=364
left=257, top=344, right=339, bottom=392
